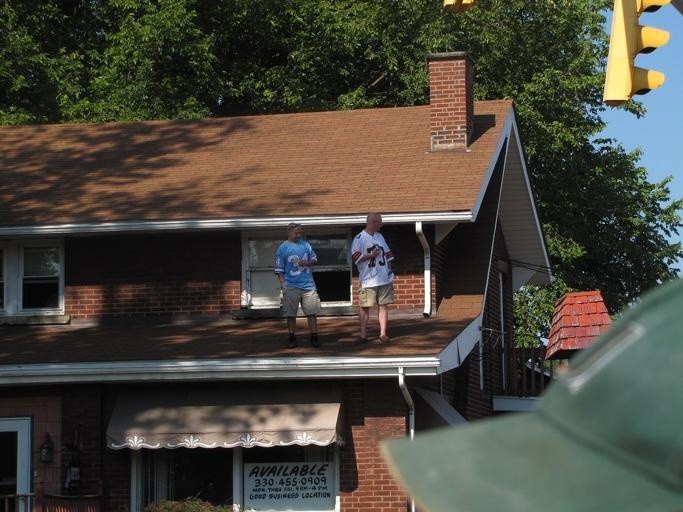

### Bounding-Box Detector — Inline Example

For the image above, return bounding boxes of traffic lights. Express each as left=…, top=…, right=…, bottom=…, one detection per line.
left=602, top=0, right=671, bottom=106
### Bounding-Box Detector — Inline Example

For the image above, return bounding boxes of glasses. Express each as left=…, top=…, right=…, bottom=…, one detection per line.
left=288, top=223, right=297, bottom=229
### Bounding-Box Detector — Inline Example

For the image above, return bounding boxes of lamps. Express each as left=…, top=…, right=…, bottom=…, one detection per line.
left=39, top=432, right=54, bottom=463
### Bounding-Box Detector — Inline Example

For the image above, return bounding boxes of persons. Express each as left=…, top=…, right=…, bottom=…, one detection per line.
left=274, top=222, right=322, bottom=349
left=348, top=212, right=396, bottom=345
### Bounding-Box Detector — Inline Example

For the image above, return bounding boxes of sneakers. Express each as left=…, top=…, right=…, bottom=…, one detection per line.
left=288, top=336, right=297, bottom=348
left=356, top=335, right=390, bottom=344
left=311, top=335, right=320, bottom=346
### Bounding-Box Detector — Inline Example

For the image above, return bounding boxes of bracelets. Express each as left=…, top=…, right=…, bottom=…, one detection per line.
left=370, top=251, right=372, bottom=257
